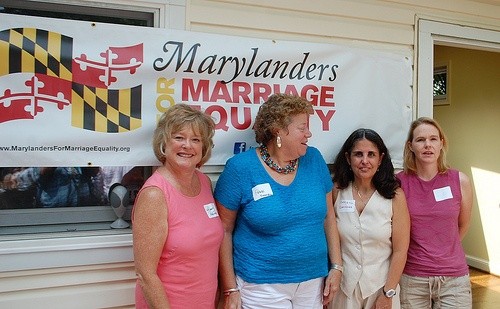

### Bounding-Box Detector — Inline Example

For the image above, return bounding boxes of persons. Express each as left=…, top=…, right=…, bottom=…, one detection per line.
left=395, top=117, right=472, bottom=309
left=214, top=93, right=343, bottom=309
left=131, top=104, right=224, bottom=309
left=332, top=128, right=410, bottom=309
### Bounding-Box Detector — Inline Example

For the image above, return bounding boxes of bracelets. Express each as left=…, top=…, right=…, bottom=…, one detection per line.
left=221, top=288, right=240, bottom=295
left=330, top=263, right=343, bottom=272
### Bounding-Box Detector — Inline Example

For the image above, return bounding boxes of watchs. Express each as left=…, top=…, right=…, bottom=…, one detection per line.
left=382, top=285, right=396, bottom=298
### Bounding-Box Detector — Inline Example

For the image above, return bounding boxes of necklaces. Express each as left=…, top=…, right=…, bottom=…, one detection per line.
left=259, top=144, right=297, bottom=175
left=354, top=182, right=375, bottom=206
